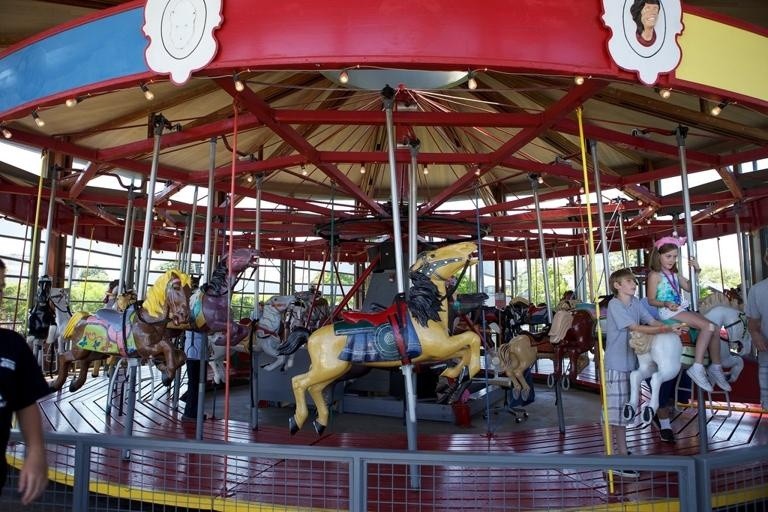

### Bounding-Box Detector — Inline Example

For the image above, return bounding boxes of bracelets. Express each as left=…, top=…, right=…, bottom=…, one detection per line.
left=660, top=325, right=665, bottom=333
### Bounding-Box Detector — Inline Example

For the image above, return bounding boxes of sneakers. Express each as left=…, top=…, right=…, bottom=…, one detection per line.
left=707, top=363, right=732, bottom=392
left=651, top=414, right=661, bottom=431
left=686, top=364, right=713, bottom=393
left=182, top=416, right=197, bottom=423
left=660, top=430, right=674, bottom=443
left=611, top=468, right=640, bottom=478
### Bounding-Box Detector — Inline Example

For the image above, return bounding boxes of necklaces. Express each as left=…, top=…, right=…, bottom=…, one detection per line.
left=663, top=271, right=681, bottom=305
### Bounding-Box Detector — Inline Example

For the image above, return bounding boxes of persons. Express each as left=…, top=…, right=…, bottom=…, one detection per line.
left=250, top=302, right=264, bottom=322
left=183, top=291, right=210, bottom=421
left=646, top=236, right=733, bottom=392
left=600, top=271, right=689, bottom=478
left=649, top=369, right=677, bottom=442
left=0, top=259, right=51, bottom=506
left=745, top=248, right=768, bottom=411
left=629, top=0, right=660, bottom=46
